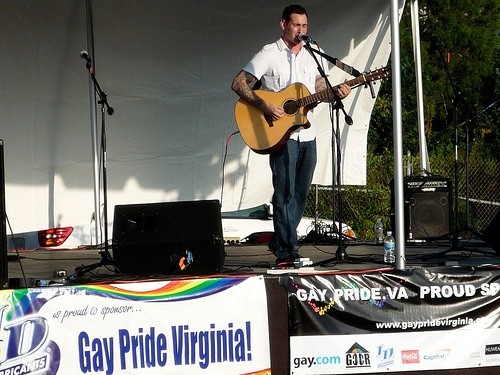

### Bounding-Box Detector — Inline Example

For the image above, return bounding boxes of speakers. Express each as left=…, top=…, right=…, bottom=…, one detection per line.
left=481, top=211, right=500, bottom=256
left=389, top=177, right=455, bottom=241
left=113, top=200, right=226, bottom=276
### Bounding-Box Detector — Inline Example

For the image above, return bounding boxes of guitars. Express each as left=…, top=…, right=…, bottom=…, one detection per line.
left=233, top=65, right=392, bottom=155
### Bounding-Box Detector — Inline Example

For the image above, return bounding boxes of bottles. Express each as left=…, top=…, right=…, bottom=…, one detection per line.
left=375, top=218, right=383, bottom=241
left=383, top=231, right=395, bottom=263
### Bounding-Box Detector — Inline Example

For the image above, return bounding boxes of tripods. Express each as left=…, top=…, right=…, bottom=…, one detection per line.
left=306, top=42, right=376, bottom=268
left=414, top=50, right=500, bottom=261
left=67, top=60, right=114, bottom=282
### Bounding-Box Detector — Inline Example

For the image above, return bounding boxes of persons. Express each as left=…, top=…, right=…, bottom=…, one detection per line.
left=230, top=4, right=351, bottom=267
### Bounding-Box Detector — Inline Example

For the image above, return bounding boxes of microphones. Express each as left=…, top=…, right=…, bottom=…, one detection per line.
left=80, top=50, right=91, bottom=61
left=298, top=33, right=317, bottom=44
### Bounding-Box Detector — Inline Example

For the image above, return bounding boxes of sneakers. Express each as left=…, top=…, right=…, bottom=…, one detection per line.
left=297, top=255, right=306, bottom=258
left=276, top=258, right=294, bottom=266
